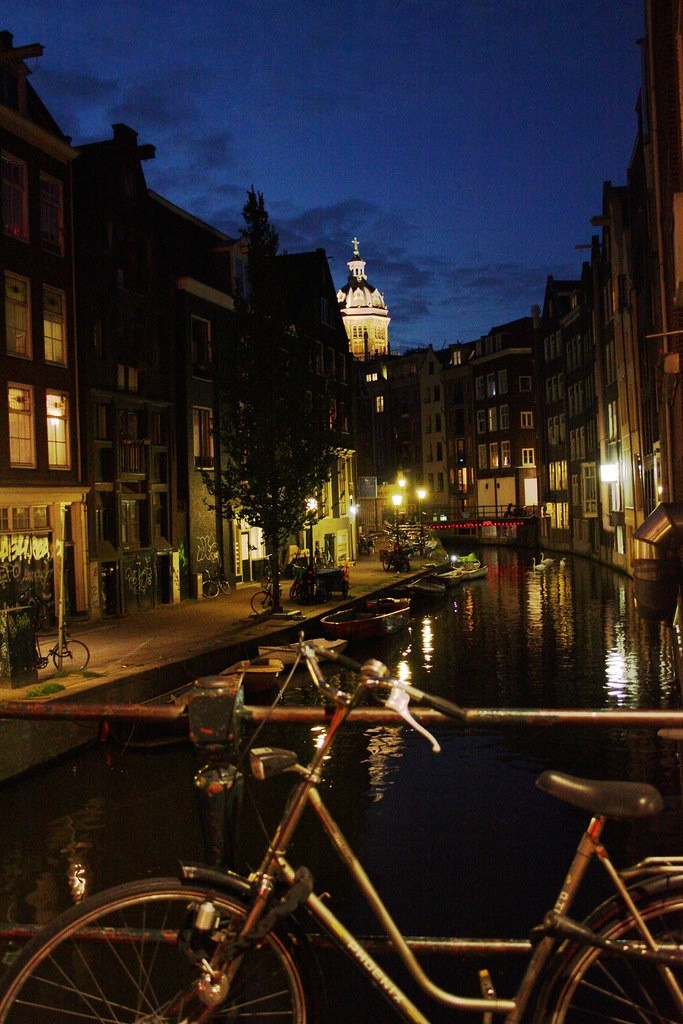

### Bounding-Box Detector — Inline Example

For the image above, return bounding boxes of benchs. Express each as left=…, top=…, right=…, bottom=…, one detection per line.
left=356, top=612, right=382, bottom=619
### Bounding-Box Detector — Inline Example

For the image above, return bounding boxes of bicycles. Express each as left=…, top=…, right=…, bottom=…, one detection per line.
left=250, top=579, right=283, bottom=615
left=0, top=630, right=683, bottom=1023
left=288, top=565, right=351, bottom=599
left=35, top=622, right=90, bottom=673
left=202, top=565, right=231, bottom=599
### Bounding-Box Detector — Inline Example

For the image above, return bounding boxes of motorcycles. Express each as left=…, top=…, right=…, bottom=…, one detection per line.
left=359, top=531, right=420, bottom=571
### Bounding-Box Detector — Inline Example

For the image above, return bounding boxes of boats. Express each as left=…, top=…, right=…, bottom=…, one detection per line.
left=164, top=638, right=348, bottom=710
left=451, top=556, right=481, bottom=571
left=320, top=597, right=411, bottom=639
left=436, top=568, right=463, bottom=589
left=461, top=565, right=489, bottom=581
left=393, top=573, right=446, bottom=597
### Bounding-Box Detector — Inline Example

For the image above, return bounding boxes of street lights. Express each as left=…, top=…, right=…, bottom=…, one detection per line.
left=418, top=491, right=425, bottom=557
left=392, top=495, right=402, bottom=571
left=305, top=498, right=317, bottom=604
left=399, top=480, right=405, bottom=510
left=350, top=506, right=356, bottom=561
